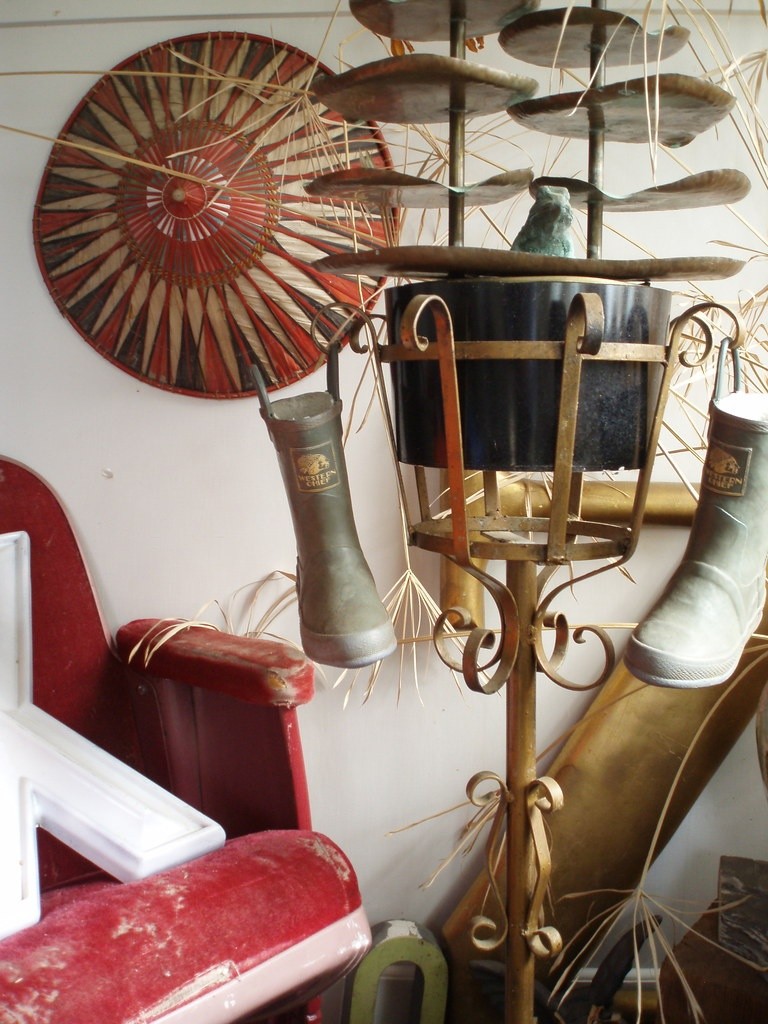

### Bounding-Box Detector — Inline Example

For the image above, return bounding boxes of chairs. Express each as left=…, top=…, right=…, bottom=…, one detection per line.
left=1, top=450, right=372, bottom=1024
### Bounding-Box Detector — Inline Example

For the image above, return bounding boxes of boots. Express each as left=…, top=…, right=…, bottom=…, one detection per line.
left=248, top=342, right=397, bottom=669
left=623, top=337, right=768, bottom=689
